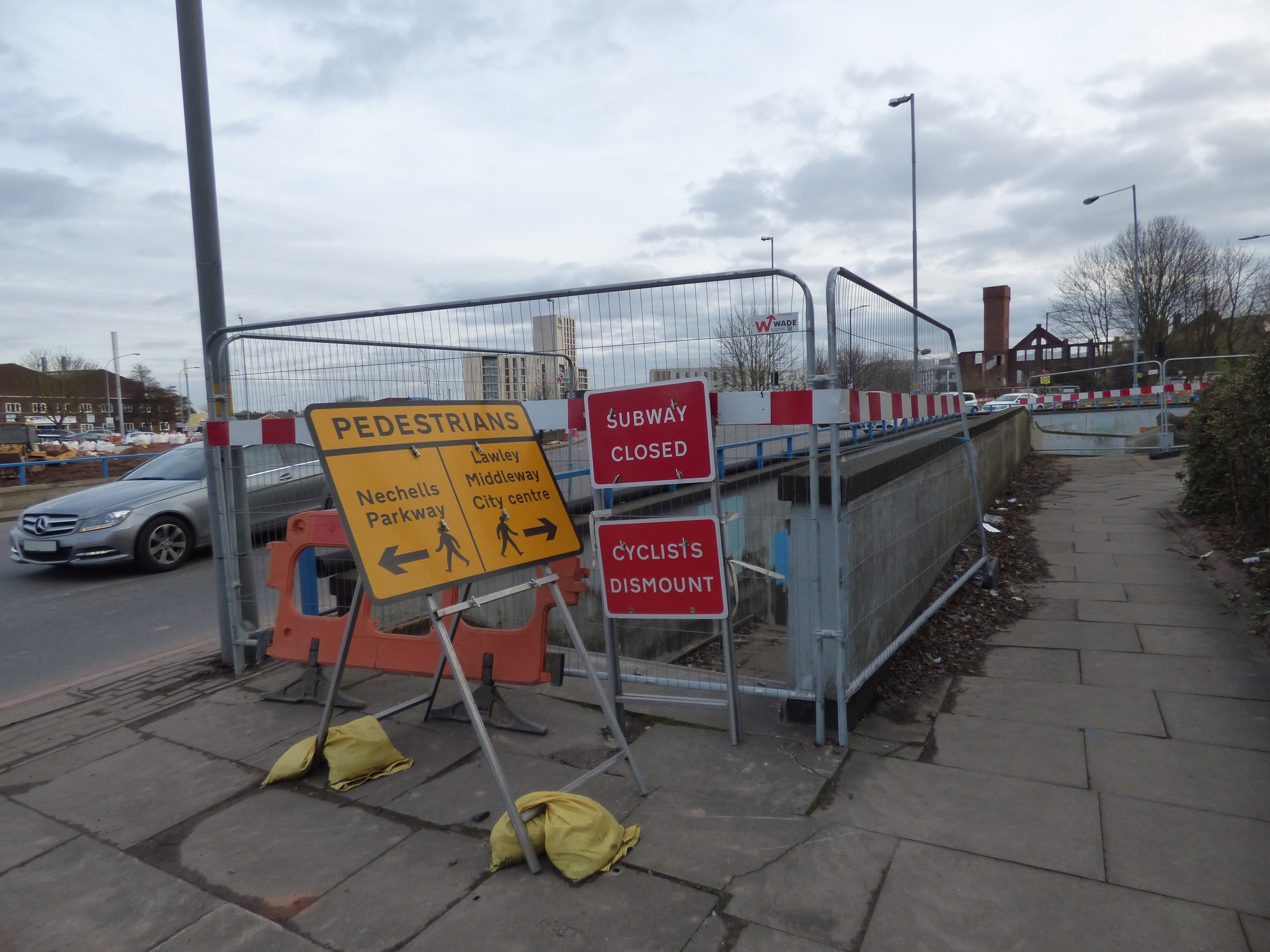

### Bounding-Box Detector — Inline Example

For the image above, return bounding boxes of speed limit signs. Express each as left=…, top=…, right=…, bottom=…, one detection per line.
left=1042, top=376, right=1049, bottom=383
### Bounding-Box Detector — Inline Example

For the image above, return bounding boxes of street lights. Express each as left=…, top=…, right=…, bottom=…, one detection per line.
left=850, top=304, right=870, bottom=378
left=106, top=353, right=140, bottom=418
left=270, top=393, right=289, bottom=414
left=410, top=365, right=445, bottom=400
left=1045, top=309, right=1067, bottom=396
left=887, top=93, right=920, bottom=396
left=1082, top=184, right=1139, bottom=388
left=179, top=366, right=199, bottom=434
left=762, top=235, right=776, bottom=390
left=238, top=316, right=250, bottom=420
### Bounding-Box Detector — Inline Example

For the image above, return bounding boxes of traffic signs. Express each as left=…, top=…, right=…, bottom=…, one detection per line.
left=302, top=400, right=583, bottom=604
left=774, top=363, right=785, bottom=369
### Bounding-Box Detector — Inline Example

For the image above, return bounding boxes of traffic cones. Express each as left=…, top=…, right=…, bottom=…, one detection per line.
left=572, top=429, right=580, bottom=437
left=561, top=429, right=569, bottom=441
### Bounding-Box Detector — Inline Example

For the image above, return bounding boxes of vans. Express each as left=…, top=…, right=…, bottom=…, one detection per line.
left=938, top=391, right=978, bottom=414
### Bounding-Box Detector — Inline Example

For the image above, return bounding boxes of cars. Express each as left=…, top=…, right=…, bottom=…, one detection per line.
left=982, top=393, right=1045, bottom=413
left=40, top=429, right=157, bottom=445
left=862, top=417, right=908, bottom=433
left=9, top=440, right=335, bottom=574
